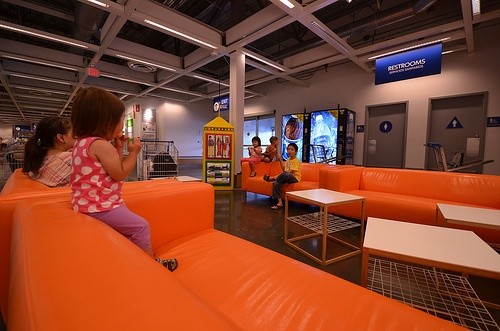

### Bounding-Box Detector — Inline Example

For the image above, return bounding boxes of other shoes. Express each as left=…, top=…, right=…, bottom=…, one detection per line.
left=237, top=171, right=242, bottom=175
left=249, top=172, right=257, bottom=177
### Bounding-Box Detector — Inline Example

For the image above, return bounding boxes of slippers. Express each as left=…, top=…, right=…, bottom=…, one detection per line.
left=271, top=204, right=283, bottom=209
left=263, top=174, right=270, bottom=182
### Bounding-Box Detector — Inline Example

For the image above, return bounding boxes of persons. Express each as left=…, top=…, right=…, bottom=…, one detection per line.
left=70, top=87, right=178, bottom=272
left=22, top=115, right=77, bottom=188
left=237, top=136, right=263, bottom=177
left=261, top=136, right=280, bottom=163
left=263, top=143, right=302, bottom=210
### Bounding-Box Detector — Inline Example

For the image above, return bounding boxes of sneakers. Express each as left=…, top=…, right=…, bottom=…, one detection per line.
left=156, top=257, right=177, bottom=272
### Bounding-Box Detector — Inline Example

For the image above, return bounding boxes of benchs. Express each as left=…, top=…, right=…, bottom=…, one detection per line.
left=0, top=160, right=500, bottom=331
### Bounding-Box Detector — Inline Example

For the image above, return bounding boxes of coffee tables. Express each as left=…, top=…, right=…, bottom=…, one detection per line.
left=434, top=201, right=500, bottom=284
left=148, top=175, right=203, bottom=182
left=284, top=188, right=366, bottom=268
left=360, top=216, right=500, bottom=331
